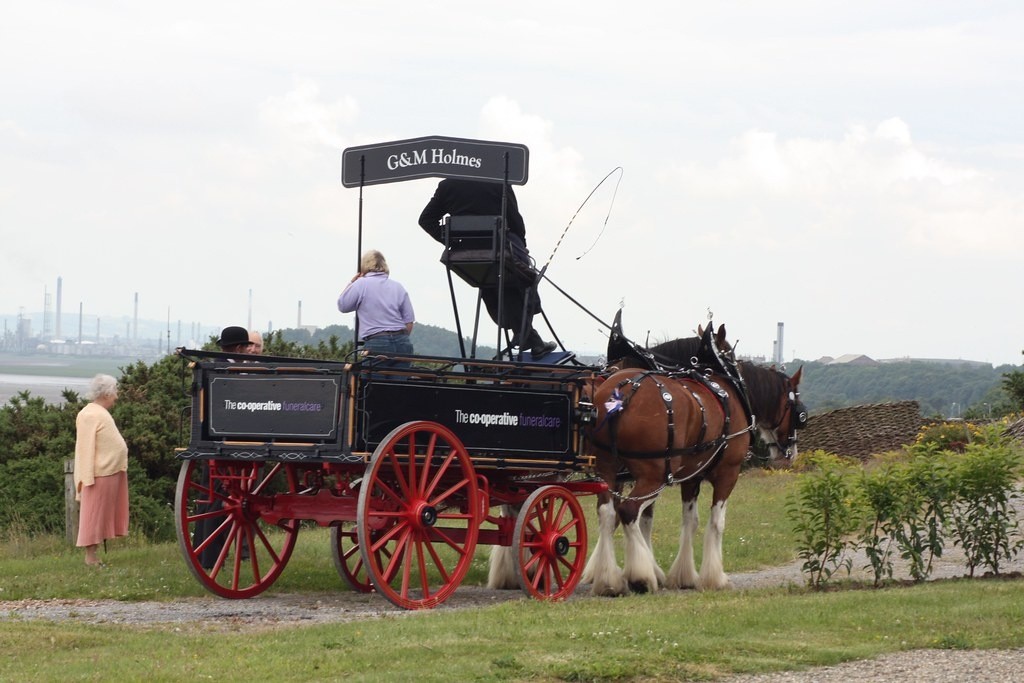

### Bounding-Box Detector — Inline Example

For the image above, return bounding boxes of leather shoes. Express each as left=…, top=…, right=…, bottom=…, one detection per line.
left=532, top=341, right=558, bottom=360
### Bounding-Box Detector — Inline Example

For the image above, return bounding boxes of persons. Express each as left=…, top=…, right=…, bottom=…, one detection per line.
left=207, top=324, right=250, bottom=376
left=418, top=177, right=559, bottom=362
left=71, top=372, right=131, bottom=574
left=237, top=332, right=267, bottom=375
left=338, top=251, right=415, bottom=368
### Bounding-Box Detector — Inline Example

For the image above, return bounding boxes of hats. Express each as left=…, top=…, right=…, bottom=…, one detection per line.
left=215, top=327, right=254, bottom=347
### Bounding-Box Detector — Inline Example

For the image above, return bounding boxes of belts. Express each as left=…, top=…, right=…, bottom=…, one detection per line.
left=367, top=330, right=408, bottom=339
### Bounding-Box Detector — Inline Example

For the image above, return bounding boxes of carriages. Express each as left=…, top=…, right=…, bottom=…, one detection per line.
left=173, top=215, right=808, bottom=609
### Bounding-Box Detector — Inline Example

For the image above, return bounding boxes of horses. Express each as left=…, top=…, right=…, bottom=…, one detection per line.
left=583, top=365, right=808, bottom=598
left=484, top=323, right=736, bottom=590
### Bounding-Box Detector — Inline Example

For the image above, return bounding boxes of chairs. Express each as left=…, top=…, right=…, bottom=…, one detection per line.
left=441, top=217, right=540, bottom=364
